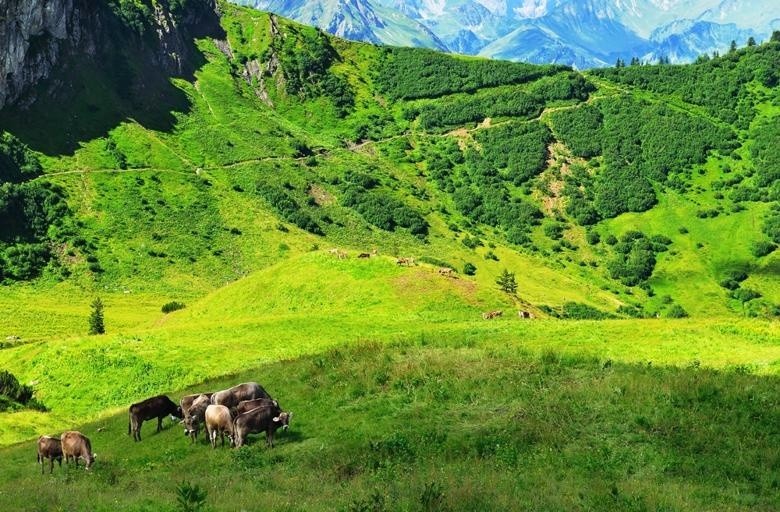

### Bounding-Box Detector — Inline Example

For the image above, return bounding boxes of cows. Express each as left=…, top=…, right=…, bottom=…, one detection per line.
left=126, top=394, right=184, bottom=442
left=483, top=310, right=502, bottom=320
left=180, top=381, right=293, bottom=450
left=519, top=310, right=536, bottom=319
left=36, top=431, right=96, bottom=475
